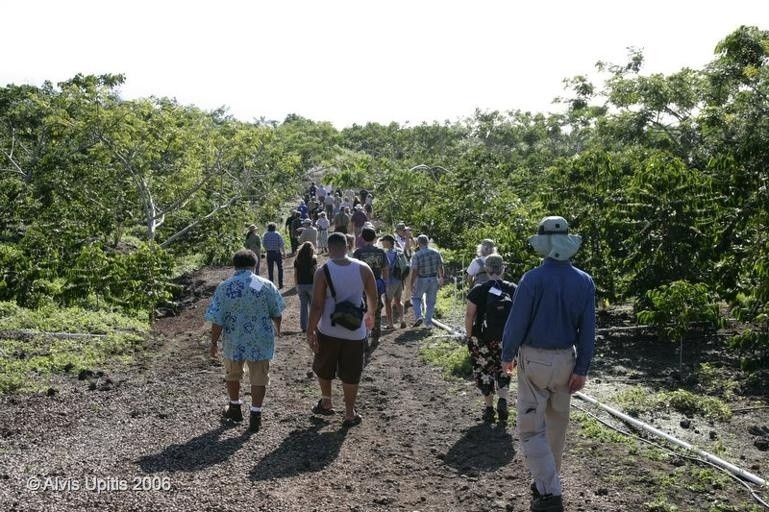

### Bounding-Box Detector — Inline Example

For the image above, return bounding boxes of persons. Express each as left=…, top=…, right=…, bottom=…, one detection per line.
left=307, top=232, right=379, bottom=424
left=204, top=249, right=287, bottom=431
left=245, top=183, right=444, bottom=337
left=466, top=255, right=518, bottom=422
left=466, top=239, right=500, bottom=289
left=500, top=215, right=597, bottom=510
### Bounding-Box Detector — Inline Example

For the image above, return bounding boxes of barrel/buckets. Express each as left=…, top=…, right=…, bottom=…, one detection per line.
left=311, top=400, right=336, bottom=416
left=343, top=409, right=362, bottom=425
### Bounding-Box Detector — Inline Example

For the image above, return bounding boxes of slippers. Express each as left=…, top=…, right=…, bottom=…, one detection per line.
left=475, top=258, right=490, bottom=283
left=484, top=292, right=513, bottom=340
left=390, top=252, right=410, bottom=281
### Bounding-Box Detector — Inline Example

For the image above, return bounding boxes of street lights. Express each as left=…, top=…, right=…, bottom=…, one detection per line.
left=330, top=300, right=367, bottom=330
left=376, top=277, right=386, bottom=294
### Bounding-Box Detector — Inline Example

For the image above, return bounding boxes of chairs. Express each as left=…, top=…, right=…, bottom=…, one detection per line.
left=220, top=401, right=243, bottom=422
left=383, top=298, right=433, bottom=336
left=248, top=405, right=262, bottom=427
left=481, top=398, right=510, bottom=422
left=530, top=481, right=563, bottom=512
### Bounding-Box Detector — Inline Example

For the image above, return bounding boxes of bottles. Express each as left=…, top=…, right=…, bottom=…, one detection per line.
left=526, top=215, right=584, bottom=262
left=379, top=222, right=409, bottom=241
left=354, top=204, right=363, bottom=209
left=302, top=212, right=325, bottom=225
left=479, top=238, right=506, bottom=268
left=248, top=224, right=258, bottom=232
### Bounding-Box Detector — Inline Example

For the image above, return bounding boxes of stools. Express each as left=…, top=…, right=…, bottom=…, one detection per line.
left=417, top=273, right=436, bottom=278
